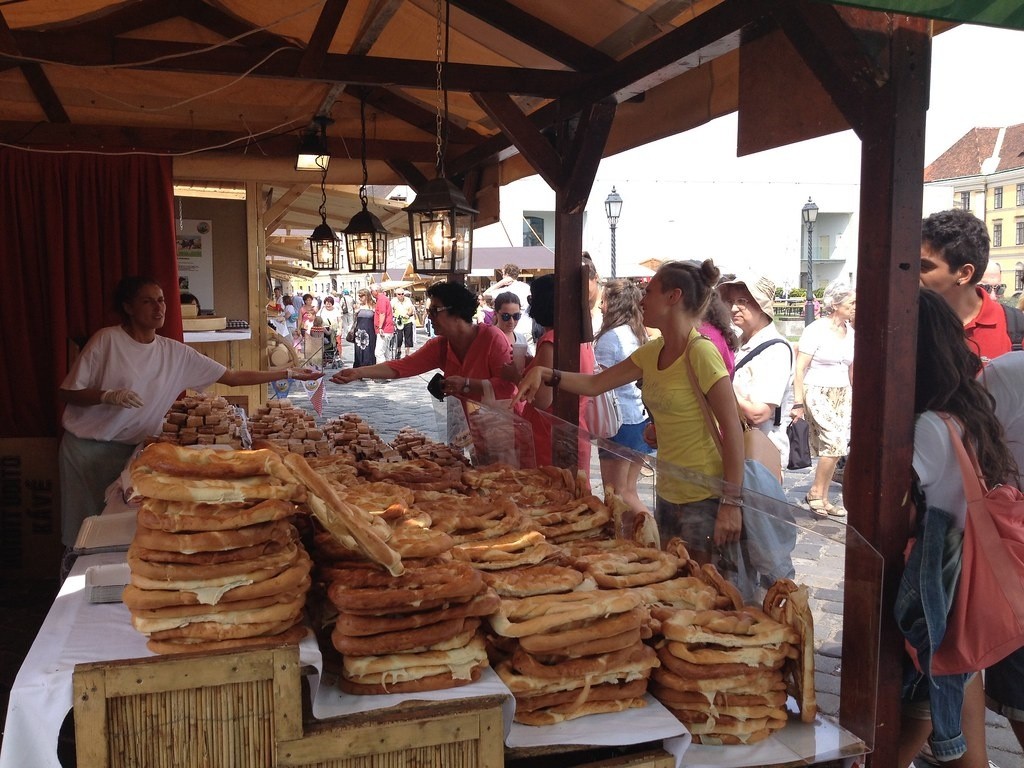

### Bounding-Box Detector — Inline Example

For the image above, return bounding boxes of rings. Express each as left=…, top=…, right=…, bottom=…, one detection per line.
left=451, top=390, right=452, bottom=392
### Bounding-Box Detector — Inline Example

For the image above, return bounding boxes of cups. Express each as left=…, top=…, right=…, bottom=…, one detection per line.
left=513, top=343, right=527, bottom=370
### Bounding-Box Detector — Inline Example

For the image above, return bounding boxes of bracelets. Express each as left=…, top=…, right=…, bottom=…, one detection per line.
left=720, top=495, right=743, bottom=507
left=287, top=369, right=292, bottom=378
left=379, top=328, right=382, bottom=329
left=544, top=369, right=562, bottom=387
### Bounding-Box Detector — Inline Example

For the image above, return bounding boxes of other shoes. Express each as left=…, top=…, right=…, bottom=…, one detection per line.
left=397, top=352, right=401, bottom=356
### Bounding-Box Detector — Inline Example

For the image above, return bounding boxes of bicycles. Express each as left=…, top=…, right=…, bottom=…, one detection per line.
left=384, top=314, right=407, bottom=360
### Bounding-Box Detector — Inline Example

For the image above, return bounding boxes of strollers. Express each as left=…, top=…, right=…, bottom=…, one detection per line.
left=322, top=318, right=346, bottom=370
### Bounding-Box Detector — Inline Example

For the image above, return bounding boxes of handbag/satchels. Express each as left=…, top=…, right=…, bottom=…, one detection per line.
left=481, top=381, right=515, bottom=454
left=743, top=427, right=783, bottom=484
left=341, top=297, right=348, bottom=314
left=289, top=312, right=299, bottom=323
left=786, top=403, right=811, bottom=470
left=346, top=321, right=355, bottom=343
left=901, top=410, right=1024, bottom=674
left=584, top=345, right=623, bottom=438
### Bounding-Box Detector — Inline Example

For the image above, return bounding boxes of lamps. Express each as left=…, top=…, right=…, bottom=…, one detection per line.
left=306, top=115, right=342, bottom=271
left=402, top=0, right=481, bottom=275
left=342, top=86, right=389, bottom=275
left=294, top=128, right=330, bottom=172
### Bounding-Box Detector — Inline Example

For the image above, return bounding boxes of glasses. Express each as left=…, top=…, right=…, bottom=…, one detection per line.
left=358, top=294, right=364, bottom=297
left=703, top=536, right=739, bottom=577
left=398, top=293, right=404, bottom=295
left=726, top=299, right=750, bottom=311
left=426, top=307, right=451, bottom=314
left=497, top=312, right=523, bottom=321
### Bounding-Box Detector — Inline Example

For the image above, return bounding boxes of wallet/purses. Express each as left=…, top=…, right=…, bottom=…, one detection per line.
left=427, top=373, right=448, bottom=402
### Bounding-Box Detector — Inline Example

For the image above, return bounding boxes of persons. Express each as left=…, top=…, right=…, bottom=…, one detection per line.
left=354, top=284, right=436, bottom=385
left=593, top=278, right=657, bottom=514
left=179, top=293, right=201, bottom=316
left=896, top=288, right=1021, bottom=768
left=508, top=258, right=745, bottom=569
left=274, top=287, right=356, bottom=358
left=483, top=264, right=536, bottom=357
left=920, top=208, right=1024, bottom=375
left=717, top=271, right=795, bottom=482
left=329, top=281, right=527, bottom=465
left=56, top=276, right=325, bottom=587
left=582, top=251, right=603, bottom=334
left=498, top=274, right=592, bottom=491
left=803, top=294, right=820, bottom=316
left=493, top=291, right=531, bottom=353
left=643, top=259, right=739, bottom=449
left=789, top=278, right=856, bottom=517
left=918, top=351, right=1024, bottom=766
left=477, top=293, right=496, bottom=324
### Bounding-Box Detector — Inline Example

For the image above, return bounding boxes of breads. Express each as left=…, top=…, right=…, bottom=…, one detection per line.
left=139, top=398, right=473, bottom=471
left=122, top=441, right=817, bottom=742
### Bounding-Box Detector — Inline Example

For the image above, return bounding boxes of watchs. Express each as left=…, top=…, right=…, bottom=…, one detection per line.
left=463, top=378, right=470, bottom=393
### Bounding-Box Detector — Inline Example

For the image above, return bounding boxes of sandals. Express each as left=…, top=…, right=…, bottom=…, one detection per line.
left=804, top=494, right=828, bottom=518
left=823, top=501, right=846, bottom=516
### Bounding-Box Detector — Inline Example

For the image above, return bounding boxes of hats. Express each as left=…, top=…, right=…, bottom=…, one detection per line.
left=714, top=273, right=776, bottom=321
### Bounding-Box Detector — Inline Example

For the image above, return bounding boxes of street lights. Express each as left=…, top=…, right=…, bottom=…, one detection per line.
left=604, top=185, right=624, bottom=280
left=802, top=195, right=819, bottom=328
